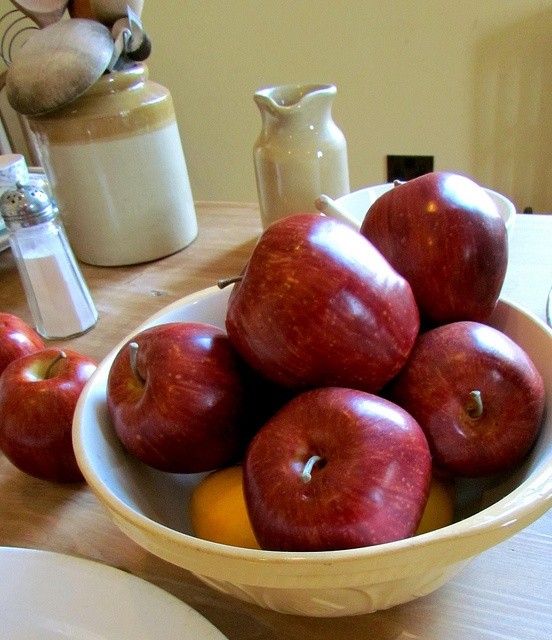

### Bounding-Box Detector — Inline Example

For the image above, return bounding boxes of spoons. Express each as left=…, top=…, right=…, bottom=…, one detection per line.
left=6, top=18, right=115, bottom=118
left=12, top=0, right=70, bottom=28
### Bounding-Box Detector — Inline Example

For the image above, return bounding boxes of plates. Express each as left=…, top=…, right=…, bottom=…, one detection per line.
left=1, top=545, right=230, bottom=640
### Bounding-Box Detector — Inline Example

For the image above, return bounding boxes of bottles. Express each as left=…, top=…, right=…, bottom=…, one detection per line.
left=252, top=83, right=351, bottom=230
left=0, top=180, right=101, bottom=341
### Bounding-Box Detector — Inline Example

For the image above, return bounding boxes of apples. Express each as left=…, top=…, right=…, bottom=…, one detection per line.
left=0, top=346, right=100, bottom=483
left=107, top=321, right=240, bottom=474
left=360, top=171, right=509, bottom=322
left=217, top=212, right=420, bottom=392
left=242, top=388, right=433, bottom=550
left=0, top=313, right=45, bottom=373
left=386, top=321, right=546, bottom=479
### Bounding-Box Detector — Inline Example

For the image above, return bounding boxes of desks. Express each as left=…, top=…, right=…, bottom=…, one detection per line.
left=0, top=201, right=551, bottom=638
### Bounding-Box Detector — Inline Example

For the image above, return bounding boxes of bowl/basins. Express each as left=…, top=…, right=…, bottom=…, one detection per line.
left=320, top=180, right=517, bottom=229
left=71, top=282, right=552, bottom=618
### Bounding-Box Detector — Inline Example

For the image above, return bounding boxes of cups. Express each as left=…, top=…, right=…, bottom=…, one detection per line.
left=0, top=153, right=30, bottom=196
left=25, top=60, right=198, bottom=267
